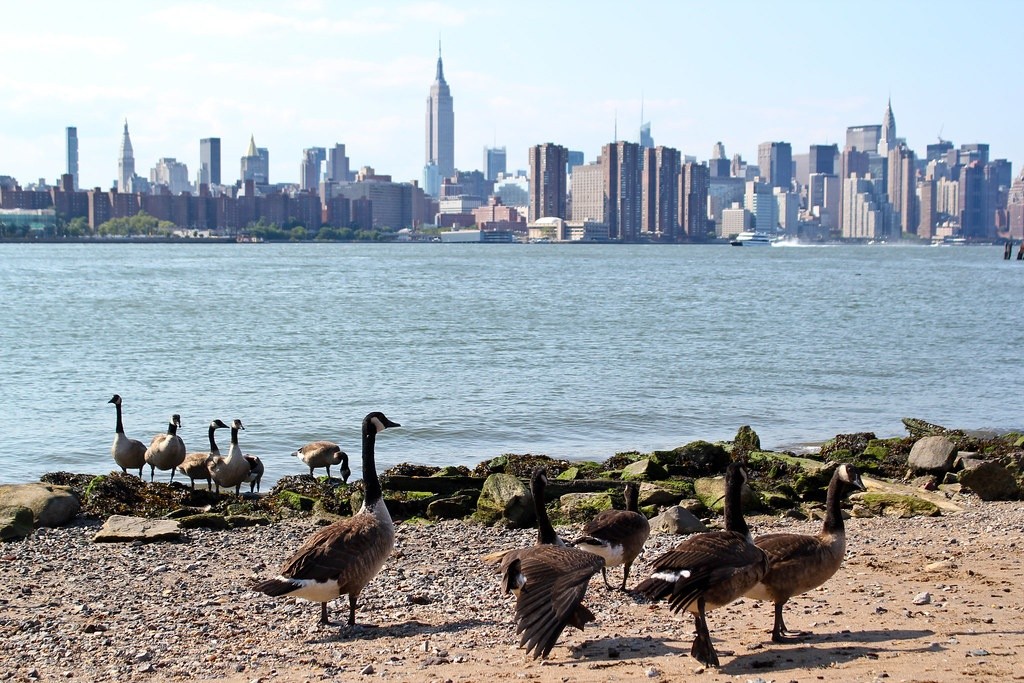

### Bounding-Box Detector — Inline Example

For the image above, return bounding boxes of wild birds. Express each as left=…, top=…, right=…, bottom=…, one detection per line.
left=175, top=418, right=230, bottom=492
left=252, top=411, right=402, bottom=629
left=570, top=480, right=651, bottom=593
left=143, top=413, right=187, bottom=486
left=235, top=453, right=264, bottom=499
left=482, top=465, right=606, bottom=663
left=290, top=441, right=351, bottom=484
left=206, top=419, right=250, bottom=500
left=108, top=393, right=148, bottom=480
left=742, top=462, right=867, bottom=643
left=627, top=463, right=769, bottom=668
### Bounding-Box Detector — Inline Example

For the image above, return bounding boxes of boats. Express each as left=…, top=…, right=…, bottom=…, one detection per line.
left=729, top=227, right=772, bottom=246
